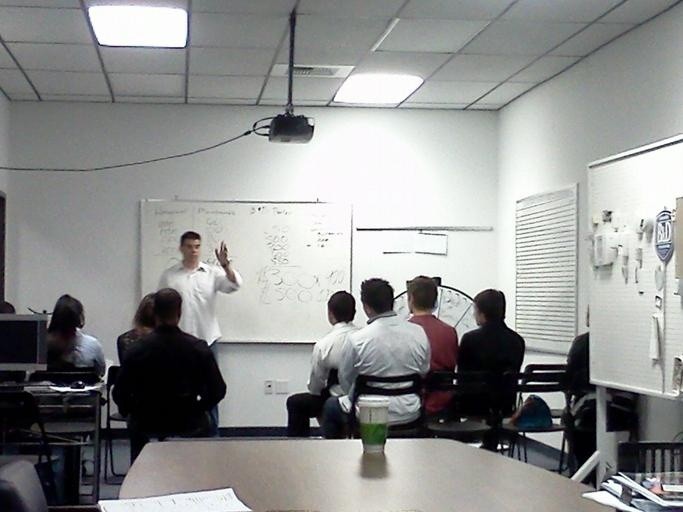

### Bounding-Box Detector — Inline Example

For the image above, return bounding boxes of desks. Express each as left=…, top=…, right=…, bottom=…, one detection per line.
left=116, top=437, right=640, bottom=512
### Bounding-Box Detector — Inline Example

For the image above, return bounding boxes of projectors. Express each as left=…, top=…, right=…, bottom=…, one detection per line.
left=268, top=113, right=314, bottom=145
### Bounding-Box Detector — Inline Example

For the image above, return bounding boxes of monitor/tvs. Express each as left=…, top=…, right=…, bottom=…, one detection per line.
left=0, top=313, right=56, bottom=386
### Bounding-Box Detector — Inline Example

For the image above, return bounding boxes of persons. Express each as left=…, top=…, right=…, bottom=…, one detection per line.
left=115, top=291, right=158, bottom=365
left=155, top=231, right=244, bottom=364
left=121, top=288, right=227, bottom=437
left=433, top=288, right=526, bottom=452
left=402, top=275, right=458, bottom=418
left=44, top=294, right=106, bottom=378
left=282, top=290, right=363, bottom=437
left=316, top=278, right=431, bottom=440
left=560, top=306, right=638, bottom=483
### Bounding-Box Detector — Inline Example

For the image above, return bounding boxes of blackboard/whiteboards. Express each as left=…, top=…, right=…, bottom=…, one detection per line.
left=139, top=198, right=353, bottom=344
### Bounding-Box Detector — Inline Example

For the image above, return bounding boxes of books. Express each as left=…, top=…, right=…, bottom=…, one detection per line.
left=581, top=469, right=683, bottom=511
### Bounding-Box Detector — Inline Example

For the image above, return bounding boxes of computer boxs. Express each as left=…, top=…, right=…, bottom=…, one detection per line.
left=78, top=446, right=94, bottom=495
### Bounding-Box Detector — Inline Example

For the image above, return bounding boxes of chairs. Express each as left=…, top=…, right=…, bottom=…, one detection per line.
left=0, top=362, right=126, bottom=511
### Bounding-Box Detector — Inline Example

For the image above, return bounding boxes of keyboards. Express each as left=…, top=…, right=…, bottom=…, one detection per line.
left=31, top=396, right=90, bottom=404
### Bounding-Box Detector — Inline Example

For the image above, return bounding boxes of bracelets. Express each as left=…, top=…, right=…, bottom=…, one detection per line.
left=220, top=259, right=232, bottom=268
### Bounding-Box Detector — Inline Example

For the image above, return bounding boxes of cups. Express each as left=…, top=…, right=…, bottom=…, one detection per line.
left=357, top=395, right=389, bottom=454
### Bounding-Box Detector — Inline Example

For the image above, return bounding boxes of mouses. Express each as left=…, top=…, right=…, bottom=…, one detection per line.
left=72, top=381, right=85, bottom=388
